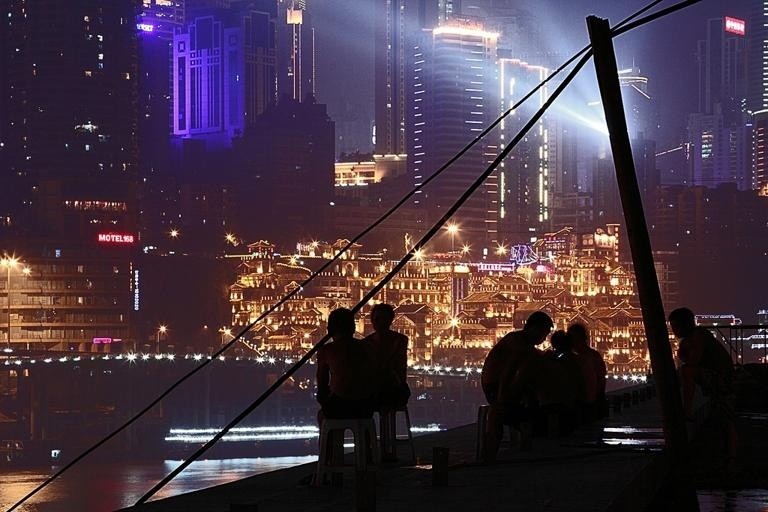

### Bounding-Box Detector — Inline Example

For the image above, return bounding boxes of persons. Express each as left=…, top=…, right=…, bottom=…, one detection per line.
left=363, top=301, right=411, bottom=412
left=313, top=308, right=377, bottom=483
left=480, top=311, right=611, bottom=471
left=668, top=307, right=739, bottom=489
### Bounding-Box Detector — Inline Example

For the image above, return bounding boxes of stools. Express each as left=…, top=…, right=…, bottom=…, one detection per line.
left=473, top=402, right=520, bottom=465
left=373, top=401, right=416, bottom=466
left=312, top=416, right=378, bottom=489
